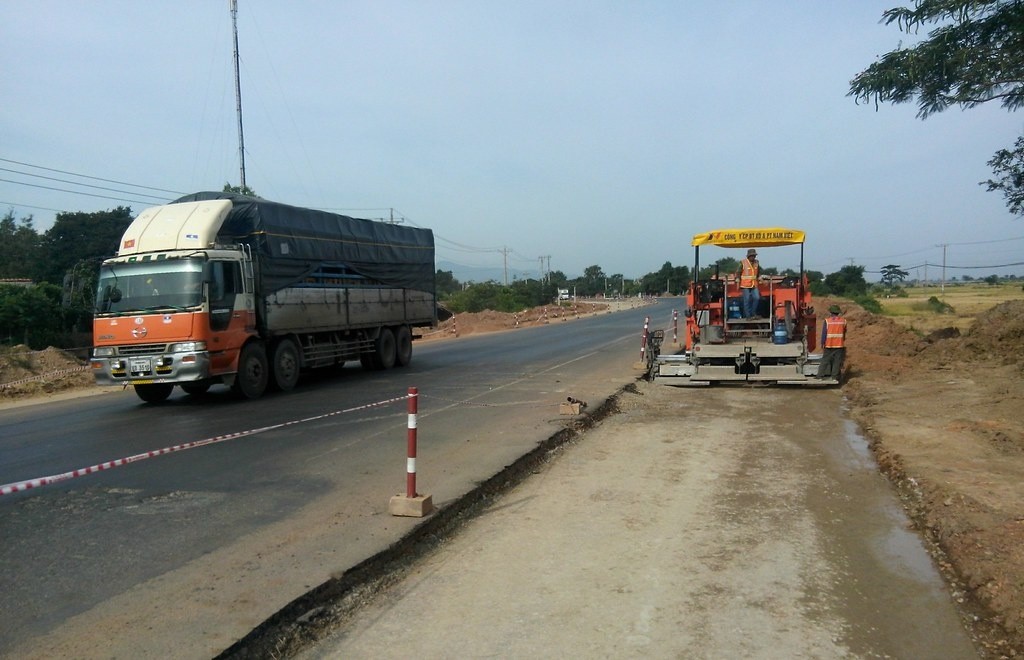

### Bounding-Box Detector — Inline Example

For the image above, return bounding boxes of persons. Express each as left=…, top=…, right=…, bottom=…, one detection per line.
left=814, top=305, right=847, bottom=381
left=735, top=249, right=763, bottom=320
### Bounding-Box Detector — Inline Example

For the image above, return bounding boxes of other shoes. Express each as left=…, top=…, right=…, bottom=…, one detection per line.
left=752, top=315, right=758, bottom=320
left=832, top=376, right=837, bottom=381
left=746, top=317, right=752, bottom=321
left=813, top=377, right=823, bottom=381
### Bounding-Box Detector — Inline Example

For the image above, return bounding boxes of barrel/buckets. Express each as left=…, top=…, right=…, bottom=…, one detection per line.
left=706, top=325, right=726, bottom=343
left=773, top=323, right=788, bottom=343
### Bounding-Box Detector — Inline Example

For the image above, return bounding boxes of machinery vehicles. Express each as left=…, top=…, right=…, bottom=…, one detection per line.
left=649, top=230, right=839, bottom=387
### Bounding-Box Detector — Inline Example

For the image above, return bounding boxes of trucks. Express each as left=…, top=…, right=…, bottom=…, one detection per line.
left=558, top=289, right=569, bottom=299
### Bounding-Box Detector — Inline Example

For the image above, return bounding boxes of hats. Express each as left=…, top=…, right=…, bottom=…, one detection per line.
left=746, top=249, right=758, bottom=257
left=827, top=305, right=843, bottom=314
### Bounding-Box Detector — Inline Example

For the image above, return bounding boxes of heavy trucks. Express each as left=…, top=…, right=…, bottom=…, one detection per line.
left=88, top=190, right=438, bottom=404
